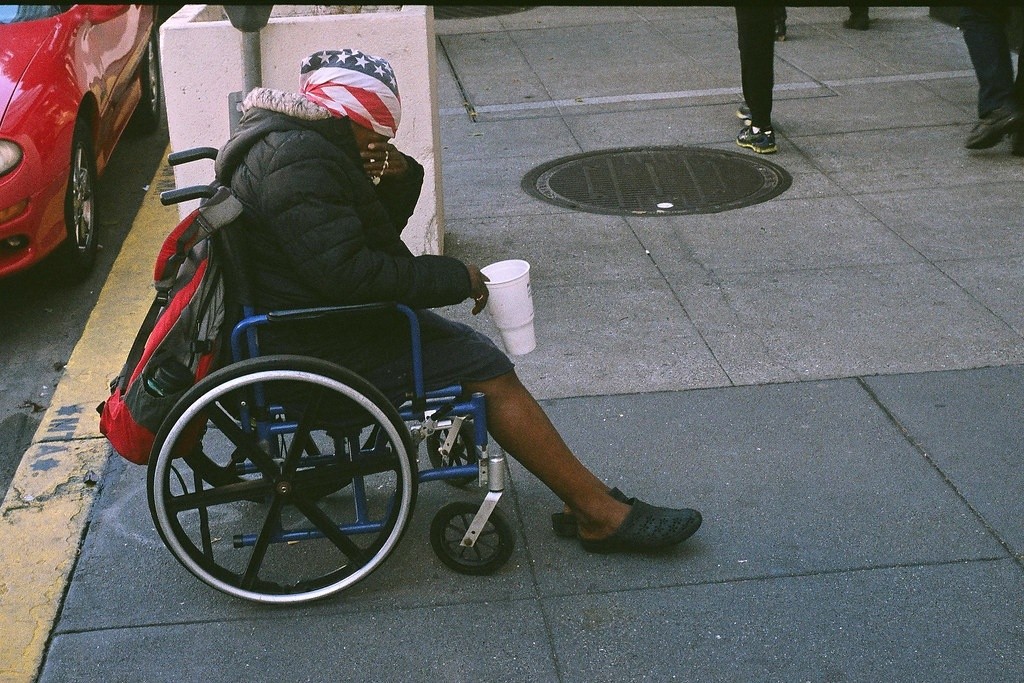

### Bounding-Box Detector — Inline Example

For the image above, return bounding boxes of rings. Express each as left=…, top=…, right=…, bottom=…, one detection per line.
left=476, top=295, right=483, bottom=300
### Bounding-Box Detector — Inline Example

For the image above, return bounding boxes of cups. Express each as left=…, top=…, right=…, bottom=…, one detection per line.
left=478, top=259, right=536, bottom=357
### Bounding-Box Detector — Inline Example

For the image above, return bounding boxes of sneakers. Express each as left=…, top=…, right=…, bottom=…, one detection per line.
left=736, top=99, right=752, bottom=120
left=736, top=126, right=779, bottom=154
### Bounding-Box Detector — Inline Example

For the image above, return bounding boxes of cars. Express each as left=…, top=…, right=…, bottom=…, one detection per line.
left=0, top=0, right=159, bottom=288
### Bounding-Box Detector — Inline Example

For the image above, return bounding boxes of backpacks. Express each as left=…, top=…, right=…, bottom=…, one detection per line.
left=96, top=185, right=243, bottom=465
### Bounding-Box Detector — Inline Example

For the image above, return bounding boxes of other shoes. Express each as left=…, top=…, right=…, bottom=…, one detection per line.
left=842, top=13, right=870, bottom=30
left=964, top=103, right=1024, bottom=149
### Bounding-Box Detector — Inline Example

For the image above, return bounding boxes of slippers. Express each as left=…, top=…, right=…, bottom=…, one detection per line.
left=551, top=487, right=630, bottom=537
left=576, top=497, right=703, bottom=554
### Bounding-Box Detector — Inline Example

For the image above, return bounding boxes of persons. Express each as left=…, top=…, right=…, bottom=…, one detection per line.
left=843, top=0, right=870, bottom=30
left=732, top=0, right=788, bottom=154
left=958, top=0, right=1024, bottom=158
left=214, top=49, right=703, bottom=553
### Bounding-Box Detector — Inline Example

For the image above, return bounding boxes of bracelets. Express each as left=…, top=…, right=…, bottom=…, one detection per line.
left=373, top=150, right=388, bottom=186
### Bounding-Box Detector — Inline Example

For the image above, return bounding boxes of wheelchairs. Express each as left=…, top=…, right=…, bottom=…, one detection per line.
left=141, top=147, right=516, bottom=607
